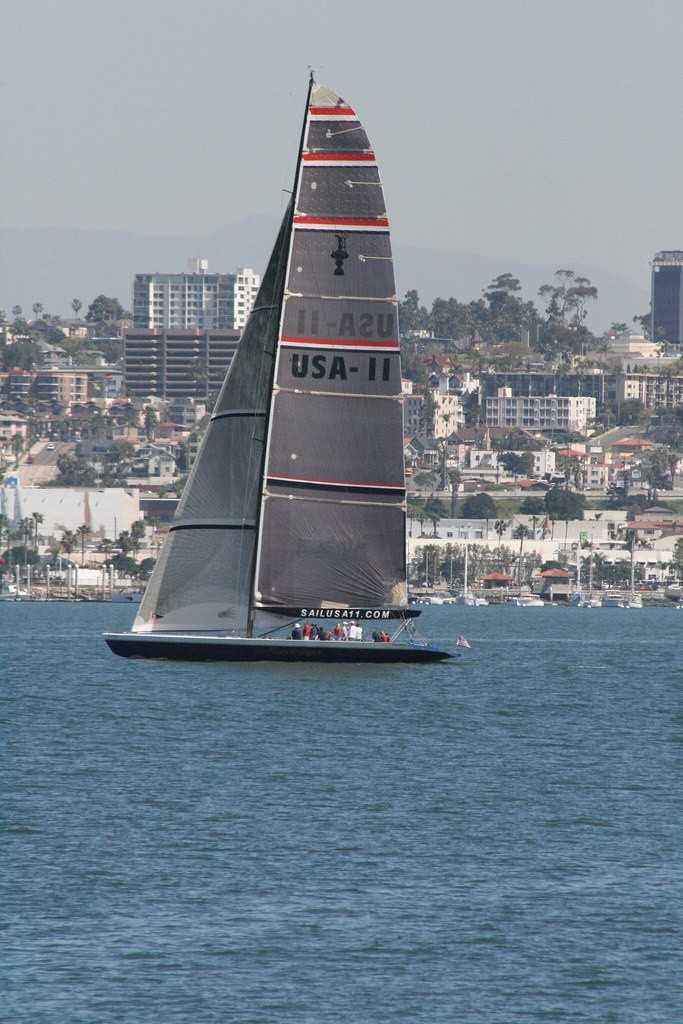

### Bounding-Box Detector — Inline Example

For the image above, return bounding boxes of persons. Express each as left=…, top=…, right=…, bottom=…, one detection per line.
left=371, top=629, right=390, bottom=643
left=290, top=621, right=363, bottom=641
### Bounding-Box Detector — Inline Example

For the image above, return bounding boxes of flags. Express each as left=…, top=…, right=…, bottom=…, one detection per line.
left=457, top=634, right=471, bottom=649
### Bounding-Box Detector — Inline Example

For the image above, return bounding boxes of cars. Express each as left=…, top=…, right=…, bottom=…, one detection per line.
left=26, top=456, right=35, bottom=464
left=45, top=554, right=77, bottom=570
left=46, top=443, right=55, bottom=450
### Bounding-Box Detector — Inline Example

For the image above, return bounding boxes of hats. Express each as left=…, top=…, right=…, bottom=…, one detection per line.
left=294, top=623, right=300, bottom=628
left=343, top=621, right=348, bottom=625
left=349, top=621, right=355, bottom=624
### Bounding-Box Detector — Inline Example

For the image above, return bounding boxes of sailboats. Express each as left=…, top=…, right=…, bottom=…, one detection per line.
left=100, top=68, right=464, bottom=664
left=404, top=534, right=683, bottom=606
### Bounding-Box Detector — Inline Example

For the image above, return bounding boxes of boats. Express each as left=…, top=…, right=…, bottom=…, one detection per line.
left=0, top=584, right=31, bottom=601
